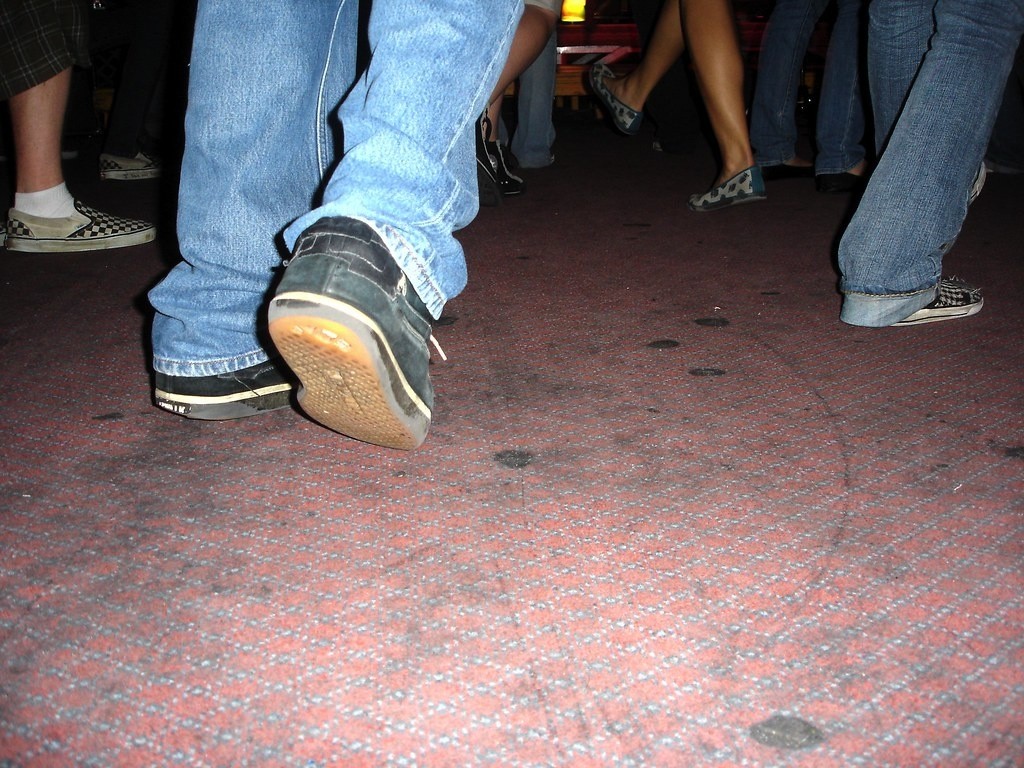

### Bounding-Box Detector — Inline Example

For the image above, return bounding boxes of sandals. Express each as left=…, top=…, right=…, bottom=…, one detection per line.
left=813, top=156, right=884, bottom=191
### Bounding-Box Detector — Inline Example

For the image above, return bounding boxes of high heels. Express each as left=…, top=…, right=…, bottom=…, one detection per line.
left=474, top=109, right=526, bottom=207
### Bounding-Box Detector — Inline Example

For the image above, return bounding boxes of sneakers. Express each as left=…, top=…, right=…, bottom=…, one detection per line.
left=153, top=357, right=298, bottom=422
left=268, top=215, right=434, bottom=449
left=964, top=159, right=988, bottom=209
left=838, top=277, right=986, bottom=325
left=98, top=152, right=166, bottom=180
left=10, top=197, right=156, bottom=253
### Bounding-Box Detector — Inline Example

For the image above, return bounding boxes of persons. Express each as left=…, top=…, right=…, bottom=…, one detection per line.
left=97, top=0, right=176, bottom=182
left=153, top=0, right=527, bottom=453
left=836, top=0, right=1023, bottom=329
left=464, top=0, right=872, bottom=214
left=0, top=0, right=157, bottom=255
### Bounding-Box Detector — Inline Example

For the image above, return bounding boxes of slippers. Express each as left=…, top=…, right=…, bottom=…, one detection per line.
left=757, top=155, right=815, bottom=181
left=589, top=62, right=644, bottom=136
left=686, top=163, right=769, bottom=213
